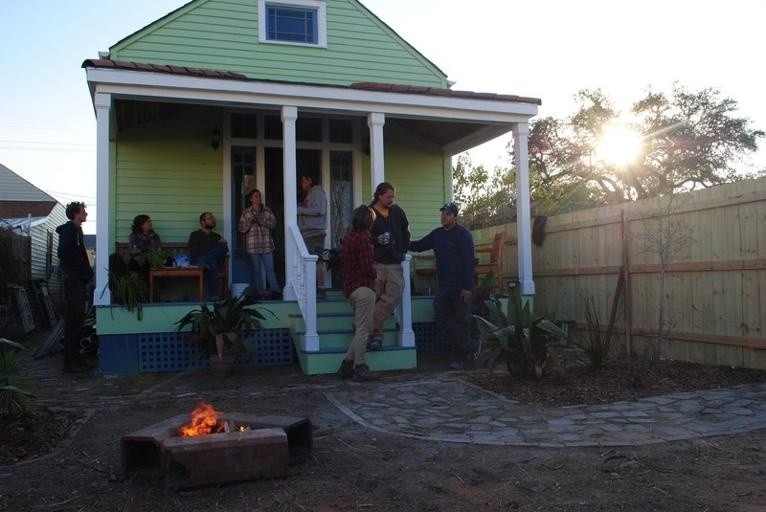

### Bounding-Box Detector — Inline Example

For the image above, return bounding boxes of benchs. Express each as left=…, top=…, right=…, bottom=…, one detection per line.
left=114, top=239, right=231, bottom=295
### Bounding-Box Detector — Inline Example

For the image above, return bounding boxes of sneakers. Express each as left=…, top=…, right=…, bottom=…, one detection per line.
left=471, top=340, right=483, bottom=362
left=68, top=359, right=95, bottom=374
left=352, top=365, right=369, bottom=380
left=336, top=359, right=353, bottom=378
left=370, top=336, right=384, bottom=350
left=449, top=359, right=464, bottom=369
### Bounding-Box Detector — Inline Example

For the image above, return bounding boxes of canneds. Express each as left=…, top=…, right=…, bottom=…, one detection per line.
left=384, top=232, right=390, bottom=243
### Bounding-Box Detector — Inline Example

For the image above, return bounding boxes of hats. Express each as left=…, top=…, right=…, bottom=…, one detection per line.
left=440, top=203, right=458, bottom=216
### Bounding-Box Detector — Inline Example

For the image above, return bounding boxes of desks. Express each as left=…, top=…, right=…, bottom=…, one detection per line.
left=146, top=262, right=204, bottom=303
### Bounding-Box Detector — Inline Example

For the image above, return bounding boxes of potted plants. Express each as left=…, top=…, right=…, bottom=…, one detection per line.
left=178, top=285, right=282, bottom=377
left=475, top=279, right=570, bottom=385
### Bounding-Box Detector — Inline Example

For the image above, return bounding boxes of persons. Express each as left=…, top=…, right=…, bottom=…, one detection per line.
left=409, top=203, right=475, bottom=370
left=55, top=201, right=92, bottom=375
left=297, top=171, right=328, bottom=301
left=335, top=204, right=383, bottom=381
left=127, top=214, right=178, bottom=304
left=187, top=212, right=229, bottom=303
left=368, top=183, right=412, bottom=350
left=239, top=189, right=279, bottom=300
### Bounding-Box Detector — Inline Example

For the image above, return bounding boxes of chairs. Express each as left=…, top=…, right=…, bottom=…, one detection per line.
left=412, top=248, right=441, bottom=298
left=472, top=229, right=508, bottom=295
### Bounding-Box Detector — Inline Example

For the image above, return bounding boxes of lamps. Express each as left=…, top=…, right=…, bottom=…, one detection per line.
left=360, top=135, right=371, bottom=157
left=211, top=121, right=221, bottom=153
